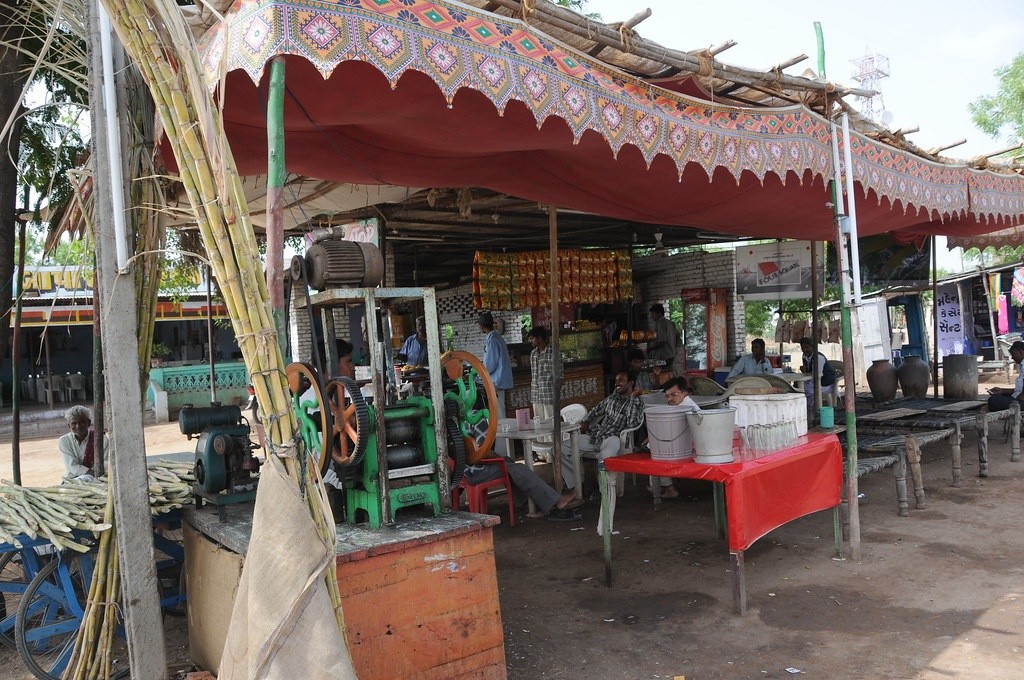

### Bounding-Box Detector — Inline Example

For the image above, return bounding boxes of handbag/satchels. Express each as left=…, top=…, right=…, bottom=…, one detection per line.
left=988, top=394, right=1010, bottom=411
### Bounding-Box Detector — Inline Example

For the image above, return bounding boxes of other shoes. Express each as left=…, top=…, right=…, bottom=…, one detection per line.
left=550, top=512, right=582, bottom=521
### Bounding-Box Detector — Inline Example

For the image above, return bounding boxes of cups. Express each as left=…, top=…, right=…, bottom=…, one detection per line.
left=533, top=416, right=540, bottom=424
left=394, top=366, right=402, bottom=385
left=738, top=421, right=799, bottom=451
left=502, top=424, right=510, bottom=433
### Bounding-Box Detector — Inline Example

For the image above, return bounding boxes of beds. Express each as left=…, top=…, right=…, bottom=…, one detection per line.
left=976, top=360, right=1013, bottom=382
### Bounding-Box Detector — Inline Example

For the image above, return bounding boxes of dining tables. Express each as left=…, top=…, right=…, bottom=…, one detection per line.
left=775, top=373, right=824, bottom=391
left=496, top=418, right=584, bottom=517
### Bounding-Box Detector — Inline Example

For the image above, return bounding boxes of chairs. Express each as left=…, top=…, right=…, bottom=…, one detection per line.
left=689, top=374, right=804, bottom=396
left=532, top=403, right=644, bottom=497
left=449, top=457, right=515, bottom=528
left=22, top=374, right=88, bottom=403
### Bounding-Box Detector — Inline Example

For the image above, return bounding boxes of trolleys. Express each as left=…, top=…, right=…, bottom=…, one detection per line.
left=0, top=506, right=195, bottom=680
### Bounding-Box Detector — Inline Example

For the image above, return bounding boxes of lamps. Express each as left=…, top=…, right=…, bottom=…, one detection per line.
left=654, top=232, right=665, bottom=250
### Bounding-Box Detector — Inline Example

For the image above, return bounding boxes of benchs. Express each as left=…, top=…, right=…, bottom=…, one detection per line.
left=651, top=397, right=1020, bottom=540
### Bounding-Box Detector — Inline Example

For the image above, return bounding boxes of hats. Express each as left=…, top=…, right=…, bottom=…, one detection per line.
left=474, top=314, right=493, bottom=325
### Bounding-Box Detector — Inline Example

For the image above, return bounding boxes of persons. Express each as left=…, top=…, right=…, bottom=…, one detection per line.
left=551, top=369, right=646, bottom=518
left=299, top=339, right=355, bottom=489
left=985, top=341, right=1024, bottom=412
left=462, top=450, right=586, bottom=513
left=647, top=303, right=676, bottom=372
left=799, top=337, right=835, bottom=387
left=476, top=312, right=514, bottom=464
left=529, top=326, right=564, bottom=463
left=58, top=405, right=109, bottom=484
left=642, top=376, right=701, bottom=499
left=727, top=338, right=774, bottom=380
left=395, top=316, right=430, bottom=367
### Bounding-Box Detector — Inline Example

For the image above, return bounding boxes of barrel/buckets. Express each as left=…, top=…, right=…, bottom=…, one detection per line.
left=642, top=405, right=694, bottom=460
left=820, top=407, right=834, bottom=428
left=685, top=407, right=737, bottom=464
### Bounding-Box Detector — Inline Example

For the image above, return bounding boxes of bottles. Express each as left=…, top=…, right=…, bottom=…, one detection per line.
left=866, top=359, right=897, bottom=403
left=570, top=351, right=581, bottom=362
left=897, top=355, right=930, bottom=399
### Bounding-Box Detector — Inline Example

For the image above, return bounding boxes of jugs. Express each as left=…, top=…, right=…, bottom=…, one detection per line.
left=516, top=408, right=530, bottom=431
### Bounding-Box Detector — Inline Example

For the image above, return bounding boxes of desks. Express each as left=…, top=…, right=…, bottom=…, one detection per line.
left=599, top=431, right=843, bottom=617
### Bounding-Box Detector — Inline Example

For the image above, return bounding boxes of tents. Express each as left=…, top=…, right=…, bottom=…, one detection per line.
left=145, top=0, right=1023, bottom=561
